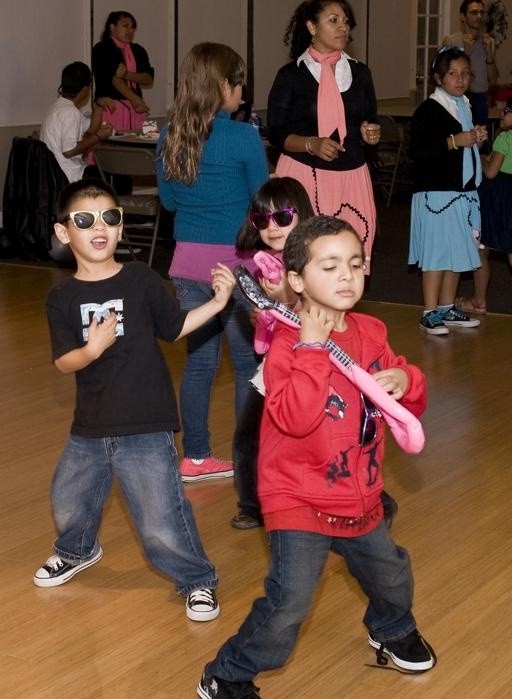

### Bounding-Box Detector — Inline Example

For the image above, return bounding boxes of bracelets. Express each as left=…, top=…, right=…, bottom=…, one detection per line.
left=305, top=136, right=316, bottom=156
left=94, top=133, right=101, bottom=143
left=449, top=134, right=458, bottom=150
left=485, top=59, right=494, bottom=64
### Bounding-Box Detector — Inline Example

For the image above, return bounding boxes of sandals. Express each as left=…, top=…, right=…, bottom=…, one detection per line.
left=454, top=297, right=487, bottom=314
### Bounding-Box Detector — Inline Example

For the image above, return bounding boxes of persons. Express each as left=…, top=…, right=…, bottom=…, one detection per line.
left=153, top=41, right=273, bottom=485
left=404, top=46, right=489, bottom=337
left=37, top=61, right=142, bottom=256
left=437, top=0, right=499, bottom=156
left=90, top=11, right=156, bottom=134
left=448, top=100, right=512, bottom=317
left=230, top=177, right=314, bottom=530
left=183, top=215, right=438, bottom=699
left=30, top=180, right=239, bottom=622
left=266, top=0, right=382, bottom=281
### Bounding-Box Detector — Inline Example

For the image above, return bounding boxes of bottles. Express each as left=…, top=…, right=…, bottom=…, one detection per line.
left=249, top=113, right=259, bottom=133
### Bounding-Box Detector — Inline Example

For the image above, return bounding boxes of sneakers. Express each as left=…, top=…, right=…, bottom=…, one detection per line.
left=439, top=305, right=481, bottom=327
left=416, top=310, right=449, bottom=335
left=33, top=544, right=103, bottom=587
left=380, top=491, right=398, bottom=528
left=115, top=243, right=141, bottom=254
left=186, top=589, right=220, bottom=621
left=179, top=456, right=234, bottom=483
left=365, top=628, right=437, bottom=674
left=231, top=514, right=258, bottom=529
left=125, top=217, right=155, bottom=228
left=198, top=662, right=261, bottom=699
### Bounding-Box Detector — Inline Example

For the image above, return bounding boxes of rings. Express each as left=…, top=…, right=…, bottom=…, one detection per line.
left=373, top=136, right=376, bottom=139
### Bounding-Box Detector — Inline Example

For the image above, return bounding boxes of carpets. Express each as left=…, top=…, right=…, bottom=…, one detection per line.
left=1, top=229, right=511, bottom=316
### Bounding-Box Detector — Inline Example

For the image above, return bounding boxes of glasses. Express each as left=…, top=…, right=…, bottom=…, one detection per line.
left=60, top=207, right=124, bottom=231
left=358, top=393, right=377, bottom=448
left=248, top=208, right=298, bottom=230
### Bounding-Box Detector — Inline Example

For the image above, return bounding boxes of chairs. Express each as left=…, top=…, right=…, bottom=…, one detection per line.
left=9, top=135, right=62, bottom=255
left=369, top=115, right=406, bottom=209
left=93, top=146, right=169, bottom=269
